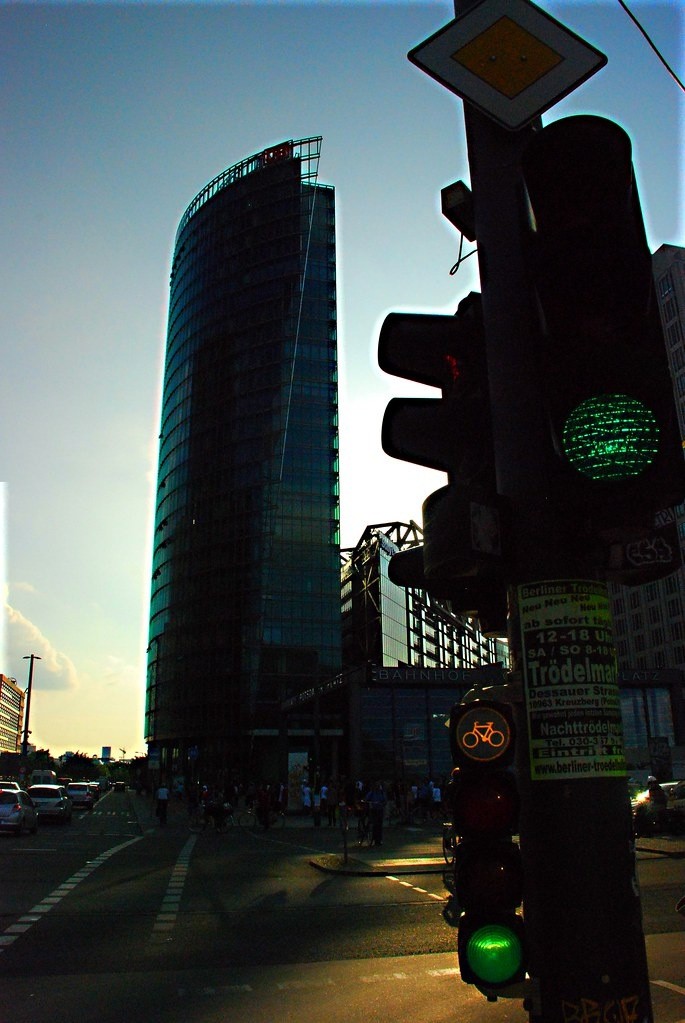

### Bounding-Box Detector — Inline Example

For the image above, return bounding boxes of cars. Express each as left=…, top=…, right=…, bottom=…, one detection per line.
left=114, top=781, right=125, bottom=792
left=89, top=781, right=115, bottom=801
left=0, top=782, right=29, bottom=793
left=632, top=782, right=685, bottom=821
left=26, top=784, right=75, bottom=825
left=57, top=777, right=74, bottom=787
left=0, top=789, right=39, bottom=836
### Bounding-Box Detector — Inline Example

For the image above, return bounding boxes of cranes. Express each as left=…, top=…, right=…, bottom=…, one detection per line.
left=120, top=748, right=127, bottom=760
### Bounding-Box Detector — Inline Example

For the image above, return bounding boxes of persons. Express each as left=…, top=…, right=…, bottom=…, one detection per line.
left=176, top=775, right=451, bottom=846
left=155, top=782, right=170, bottom=825
left=649, top=776, right=672, bottom=836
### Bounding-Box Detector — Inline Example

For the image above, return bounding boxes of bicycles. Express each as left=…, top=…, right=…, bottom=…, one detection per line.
left=182, top=803, right=234, bottom=833
left=355, top=798, right=383, bottom=848
left=237, top=801, right=287, bottom=832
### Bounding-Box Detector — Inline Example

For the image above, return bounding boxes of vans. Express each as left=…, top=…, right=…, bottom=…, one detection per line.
left=29, top=769, right=58, bottom=787
left=67, top=782, right=95, bottom=810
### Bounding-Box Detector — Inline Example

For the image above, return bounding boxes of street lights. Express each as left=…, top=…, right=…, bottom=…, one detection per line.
left=22, top=654, right=42, bottom=773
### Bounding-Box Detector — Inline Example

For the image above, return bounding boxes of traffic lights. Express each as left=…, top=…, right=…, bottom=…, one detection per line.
left=375, top=290, right=503, bottom=644
left=529, top=105, right=684, bottom=520
left=448, top=702, right=521, bottom=1001
